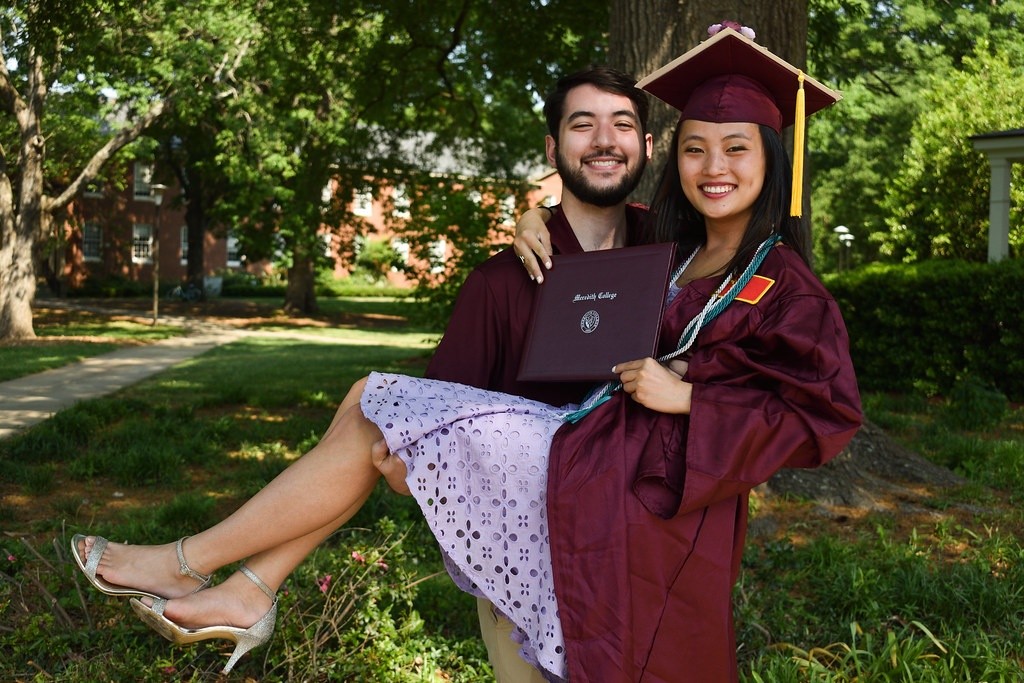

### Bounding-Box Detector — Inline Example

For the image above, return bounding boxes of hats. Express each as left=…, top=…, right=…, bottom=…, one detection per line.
left=632, top=20, right=844, bottom=221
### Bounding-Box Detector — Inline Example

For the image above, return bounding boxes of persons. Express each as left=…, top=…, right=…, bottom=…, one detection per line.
left=72, top=21, right=865, bottom=683
left=370, top=67, right=653, bottom=683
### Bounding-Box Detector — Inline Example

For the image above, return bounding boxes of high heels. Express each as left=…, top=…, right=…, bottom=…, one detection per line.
left=129, top=564, right=278, bottom=675
left=71, top=531, right=216, bottom=604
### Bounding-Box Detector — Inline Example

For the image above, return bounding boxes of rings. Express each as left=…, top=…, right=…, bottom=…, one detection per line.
left=520, top=256, right=525, bottom=263
